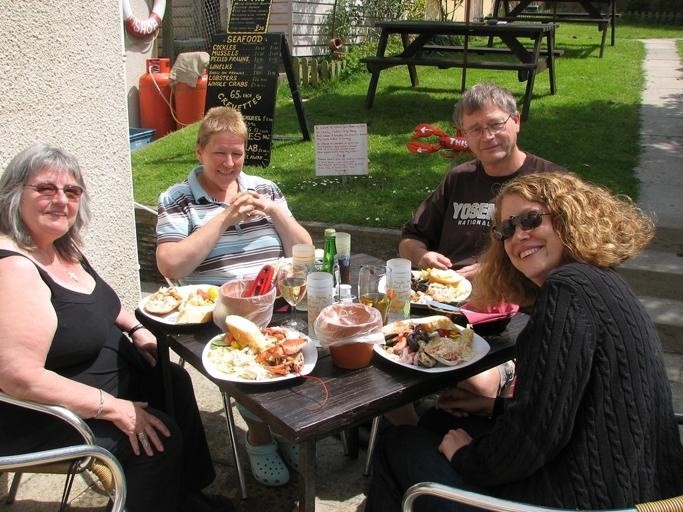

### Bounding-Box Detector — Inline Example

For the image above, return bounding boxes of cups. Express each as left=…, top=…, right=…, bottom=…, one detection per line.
left=384, top=258, right=411, bottom=322
left=276, top=228, right=355, bottom=347
left=328, top=38, right=343, bottom=50
left=218, top=278, right=276, bottom=329
left=358, top=265, right=379, bottom=308
left=323, top=303, right=382, bottom=369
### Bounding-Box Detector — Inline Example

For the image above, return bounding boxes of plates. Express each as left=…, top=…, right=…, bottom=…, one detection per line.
left=377, top=269, right=472, bottom=308
left=137, top=283, right=222, bottom=327
left=201, top=327, right=320, bottom=386
left=372, top=315, right=489, bottom=374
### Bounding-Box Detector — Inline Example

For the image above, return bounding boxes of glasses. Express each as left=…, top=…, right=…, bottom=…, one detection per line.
left=460, top=113, right=511, bottom=137
left=23, top=182, right=84, bottom=202
left=492, top=210, right=550, bottom=241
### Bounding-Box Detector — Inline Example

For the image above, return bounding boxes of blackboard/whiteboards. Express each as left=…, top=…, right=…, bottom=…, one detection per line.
left=204, top=0, right=282, bottom=169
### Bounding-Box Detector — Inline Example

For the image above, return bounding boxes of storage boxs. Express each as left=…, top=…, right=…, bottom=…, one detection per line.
left=129, top=128, right=156, bottom=153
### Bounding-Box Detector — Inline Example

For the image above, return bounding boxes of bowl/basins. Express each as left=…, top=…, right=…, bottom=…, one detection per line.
left=427, top=302, right=511, bottom=336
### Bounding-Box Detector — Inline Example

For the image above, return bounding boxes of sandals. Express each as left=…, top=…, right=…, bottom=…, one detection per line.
left=244, top=430, right=290, bottom=487
left=270, top=430, right=300, bottom=473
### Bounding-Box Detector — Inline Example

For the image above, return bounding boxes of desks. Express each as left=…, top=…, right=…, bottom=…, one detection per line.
left=132, top=253, right=529, bottom=512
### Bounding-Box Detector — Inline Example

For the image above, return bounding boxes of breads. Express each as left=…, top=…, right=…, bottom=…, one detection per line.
left=225, top=313, right=263, bottom=350
left=423, top=266, right=460, bottom=287
left=176, top=306, right=213, bottom=325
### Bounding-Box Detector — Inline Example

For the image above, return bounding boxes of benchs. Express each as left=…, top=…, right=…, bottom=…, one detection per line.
left=359, top=0, right=621, bottom=121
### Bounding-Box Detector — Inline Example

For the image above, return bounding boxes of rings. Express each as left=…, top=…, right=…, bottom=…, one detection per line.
left=138, top=432, right=150, bottom=447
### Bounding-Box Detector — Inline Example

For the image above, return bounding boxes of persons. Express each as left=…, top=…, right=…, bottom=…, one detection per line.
left=1, top=142, right=217, bottom=512
left=383, top=82, right=570, bottom=427
left=155, top=106, right=313, bottom=487
left=365, top=171, right=683, bottom=512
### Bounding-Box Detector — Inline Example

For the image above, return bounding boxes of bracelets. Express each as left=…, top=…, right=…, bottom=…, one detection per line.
left=93, top=389, right=104, bottom=418
left=128, top=324, right=144, bottom=337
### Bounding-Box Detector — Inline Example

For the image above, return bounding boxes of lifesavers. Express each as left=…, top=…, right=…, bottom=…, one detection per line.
left=124, top=0, right=168, bottom=42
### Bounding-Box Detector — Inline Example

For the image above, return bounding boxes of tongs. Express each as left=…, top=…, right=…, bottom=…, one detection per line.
left=246, top=264, right=274, bottom=298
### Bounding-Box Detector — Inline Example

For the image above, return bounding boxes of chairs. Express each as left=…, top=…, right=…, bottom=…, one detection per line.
left=1, top=393, right=125, bottom=512
left=363, top=262, right=533, bottom=482
left=401, top=412, right=682, bottom=511
left=161, top=272, right=347, bottom=499
